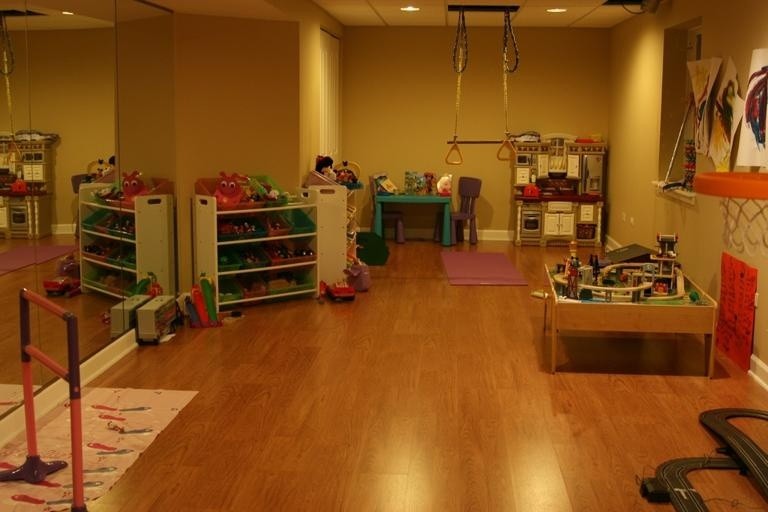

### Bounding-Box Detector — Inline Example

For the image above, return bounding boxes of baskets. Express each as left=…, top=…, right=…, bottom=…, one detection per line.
left=218, top=278, right=243, bottom=301
left=283, top=209, right=315, bottom=234
left=335, top=160, right=361, bottom=183
left=237, top=275, right=267, bottom=298
left=195, top=178, right=266, bottom=210
left=216, top=245, right=242, bottom=272
left=263, top=268, right=315, bottom=295
left=305, top=171, right=350, bottom=195
left=81, top=209, right=136, bottom=239
left=250, top=173, right=287, bottom=207
left=261, top=239, right=317, bottom=266
left=237, top=243, right=269, bottom=269
left=93, top=177, right=169, bottom=210
left=82, top=268, right=137, bottom=297
left=82, top=241, right=136, bottom=270
left=217, top=216, right=268, bottom=241
left=256, top=212, right=292, bottom=236
left=91, top=170, right=114, bottom=183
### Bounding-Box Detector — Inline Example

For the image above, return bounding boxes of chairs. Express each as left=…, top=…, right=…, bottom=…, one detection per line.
left=367, top=175, right=406, bottom=244
left=432, top=175, right=482, bottom=245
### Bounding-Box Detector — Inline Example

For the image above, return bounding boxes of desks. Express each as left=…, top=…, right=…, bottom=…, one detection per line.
left=529, top=261, right=718, bottom=377
left=372, top=192, right=447, bottom=249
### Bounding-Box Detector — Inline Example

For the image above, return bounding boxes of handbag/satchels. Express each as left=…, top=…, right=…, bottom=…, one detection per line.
left=343, top=263, right=370, bottom=292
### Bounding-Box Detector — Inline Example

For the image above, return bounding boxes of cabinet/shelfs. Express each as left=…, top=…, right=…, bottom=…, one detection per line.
left=187, top=186, right=321, bottom=313
left=309, top=160, right=361, bottom=284
left=509, top=133, right=608, bottom=252
left=0, top=127, right=59, bottom=241
left=74, top=179, right=173, bottom=301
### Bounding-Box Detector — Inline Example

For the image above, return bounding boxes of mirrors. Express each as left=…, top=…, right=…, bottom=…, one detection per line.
left=1, top=0, right=175, bottom=414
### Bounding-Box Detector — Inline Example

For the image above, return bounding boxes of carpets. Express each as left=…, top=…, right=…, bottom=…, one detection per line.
left=0, top=388, right=198, bottom=512
left=352, top=231, right=388, bottom=266
left=0, top=384, right=39, bottom=415
left=438, top=251, right=528, bottom=287
left=0, top=235, right=76, bottom=279
